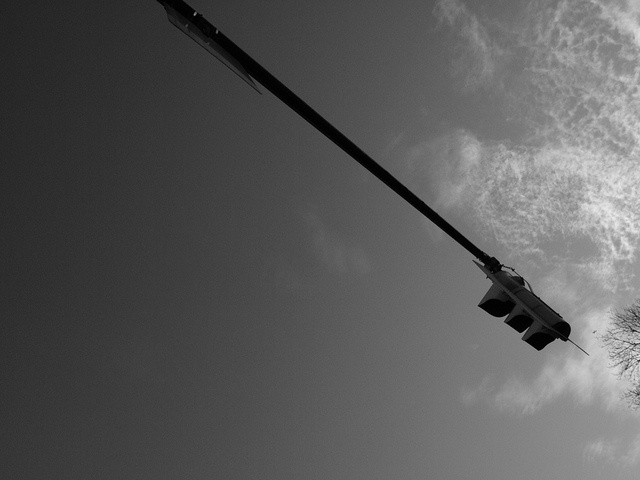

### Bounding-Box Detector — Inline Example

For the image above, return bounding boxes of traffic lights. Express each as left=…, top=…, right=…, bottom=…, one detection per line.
left=477, top=263, right=572, bottom=350
